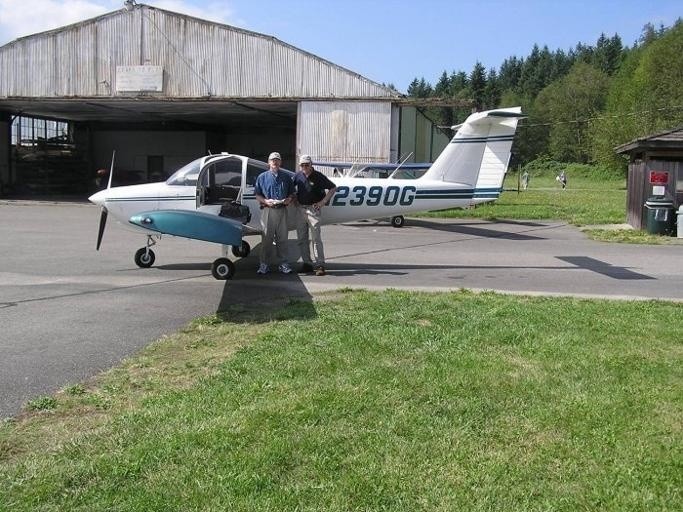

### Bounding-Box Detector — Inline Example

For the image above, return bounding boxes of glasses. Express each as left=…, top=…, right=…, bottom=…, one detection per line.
left=300, top=164, right=310, bottom=167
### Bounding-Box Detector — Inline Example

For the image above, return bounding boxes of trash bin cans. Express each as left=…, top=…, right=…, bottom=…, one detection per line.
left=644, top=195, right=678, bottom=235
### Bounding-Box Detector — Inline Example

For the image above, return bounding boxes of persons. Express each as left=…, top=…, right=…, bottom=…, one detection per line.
left=559, top=170, right=568, bottom=190
left=290, top=156, right=337, bottom=275
left=254, top=152, right=295, bottom=275
left=522, top=170, right=530, bottom=190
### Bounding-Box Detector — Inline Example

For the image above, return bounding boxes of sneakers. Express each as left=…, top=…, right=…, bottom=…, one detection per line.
left=278, top=264, right=292, bottom=274
left=316, top=265, right=325, bottom=275
left=257, top=263, right=270, bottom=274
left=296, top=263, right=313, bottom=273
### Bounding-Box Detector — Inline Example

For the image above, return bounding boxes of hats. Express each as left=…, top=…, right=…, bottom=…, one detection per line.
left=298, top=155, right=311, bottom=165
left=269, top=152, right=281, bottom=160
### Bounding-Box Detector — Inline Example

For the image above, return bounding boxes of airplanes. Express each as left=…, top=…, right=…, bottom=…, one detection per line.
left=88, top=105, right=530, bottom=280
